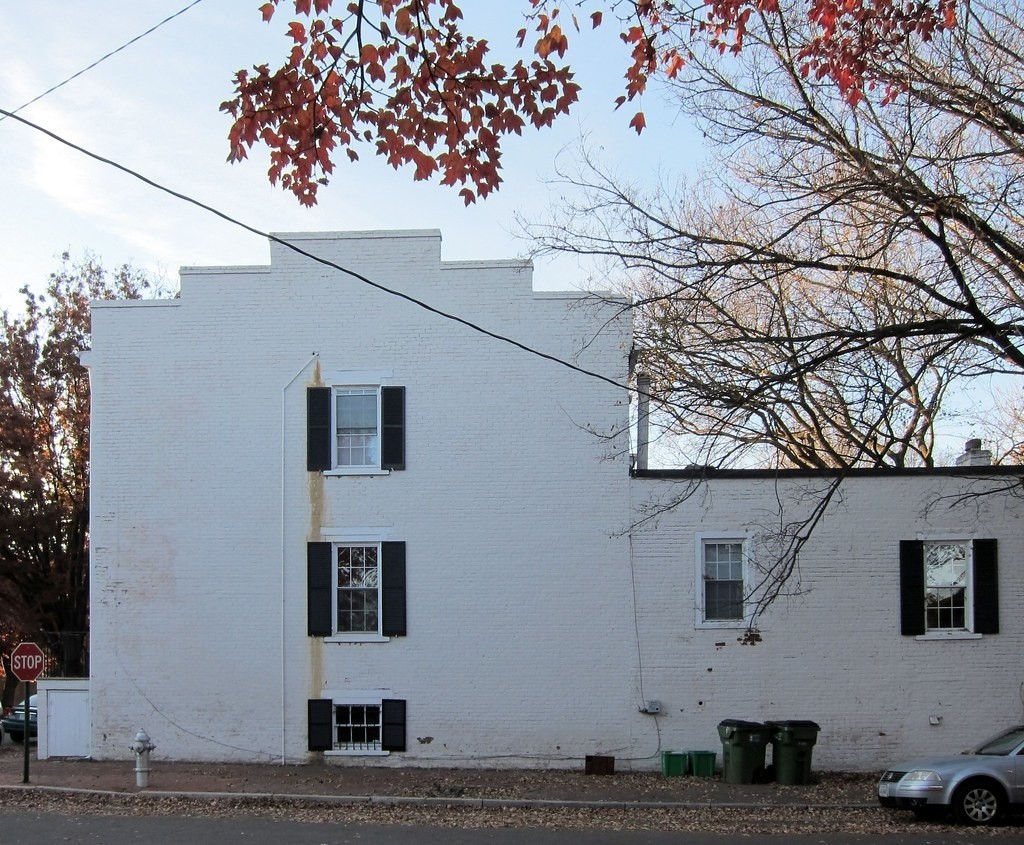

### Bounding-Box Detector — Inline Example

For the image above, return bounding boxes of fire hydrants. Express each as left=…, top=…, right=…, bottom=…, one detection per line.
left=127, top=728, right=158, bottom=788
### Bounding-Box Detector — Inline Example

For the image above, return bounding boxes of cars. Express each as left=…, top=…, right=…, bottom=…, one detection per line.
left=874, top=723, right=1024, bottom=826
left=1, top=693, right=37, bottom=742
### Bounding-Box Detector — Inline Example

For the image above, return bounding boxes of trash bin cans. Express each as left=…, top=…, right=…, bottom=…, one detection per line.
left=717, top=719, right=776, bottom=784
left=764, top=720, right=821, bottom=784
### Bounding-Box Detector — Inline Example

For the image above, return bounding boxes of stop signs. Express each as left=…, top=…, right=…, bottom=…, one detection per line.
left=11, top=642, right=45, bottom=682
